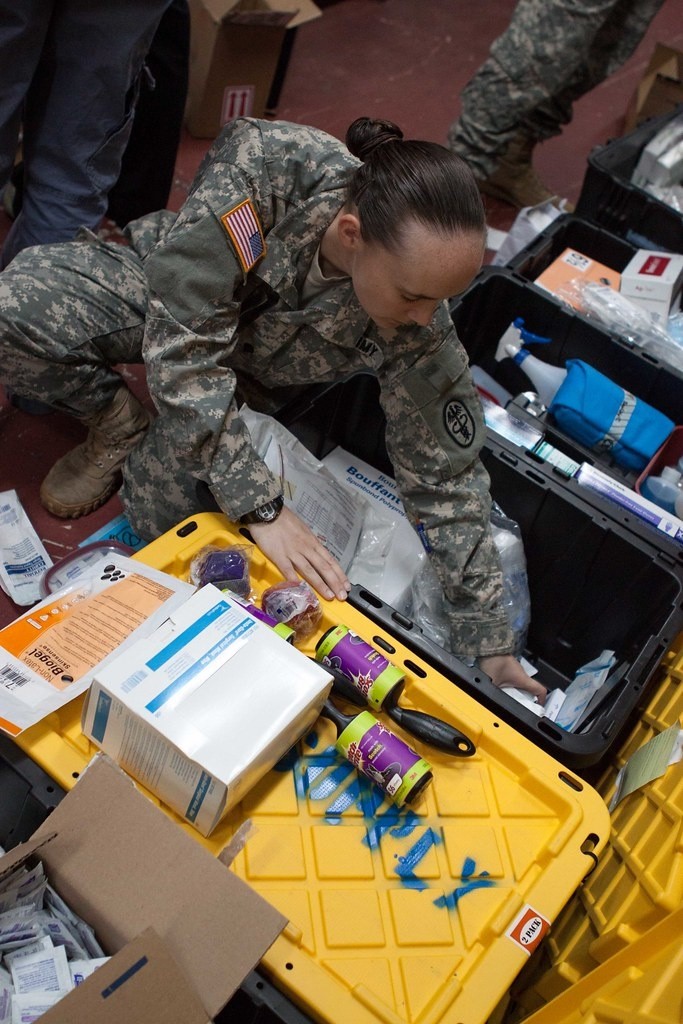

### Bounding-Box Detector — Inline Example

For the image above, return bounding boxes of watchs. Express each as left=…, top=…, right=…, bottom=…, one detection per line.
left=236, top=496, right=284, bottom=525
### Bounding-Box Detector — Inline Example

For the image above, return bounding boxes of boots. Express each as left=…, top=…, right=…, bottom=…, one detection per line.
left=470, top=116, right=575, bottom=216
left=39, top=385, right=155, bottom=519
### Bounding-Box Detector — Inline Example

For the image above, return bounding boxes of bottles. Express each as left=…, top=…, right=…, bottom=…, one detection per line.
left=638, top=466, right=682, bottom=517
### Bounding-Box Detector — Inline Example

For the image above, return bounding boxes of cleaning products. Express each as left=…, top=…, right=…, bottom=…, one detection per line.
left=495, top=318, right=567, bottom=408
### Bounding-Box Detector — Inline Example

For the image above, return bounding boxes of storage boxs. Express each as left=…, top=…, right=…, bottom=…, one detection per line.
left=80, top=581, right=335, bottom=836
left=437, top=264, right=682, bottom=562
left=576, top=105, right=682, bottom=257
left=0, top=749, right=291, bottom=1024
left=504, top=210, right=682, bottom=377
left=320, top=445, right=431, bottom=616
left=251, top=371, right=682, bottom=770
left=624, top=40, right=683, bottom=136
left=1, top=510, right=611, bottom=1024
left=183, top=0, right=323, bottom=140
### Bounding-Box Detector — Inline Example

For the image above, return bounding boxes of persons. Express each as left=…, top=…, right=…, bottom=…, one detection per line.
left=0, top=0, right=176, bottom=407
left=0, top=115, right=548, bottom=705
left=444, top=0, right=663, bottom=211
